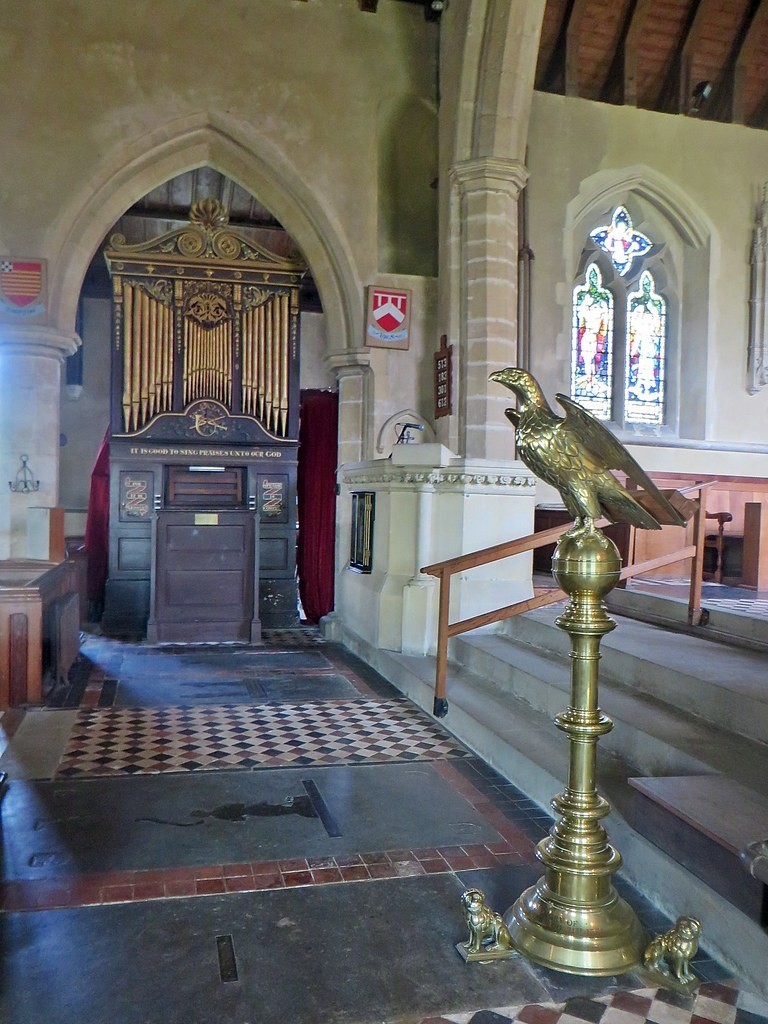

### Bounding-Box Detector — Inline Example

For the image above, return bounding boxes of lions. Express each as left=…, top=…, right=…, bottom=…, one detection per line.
left=644, top=916, right=701, bottom=984
left=460, top=888, right=511, bottom=953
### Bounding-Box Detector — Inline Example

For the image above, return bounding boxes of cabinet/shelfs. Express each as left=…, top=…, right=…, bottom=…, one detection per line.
left=1, top=558, right=81, bottom=713
